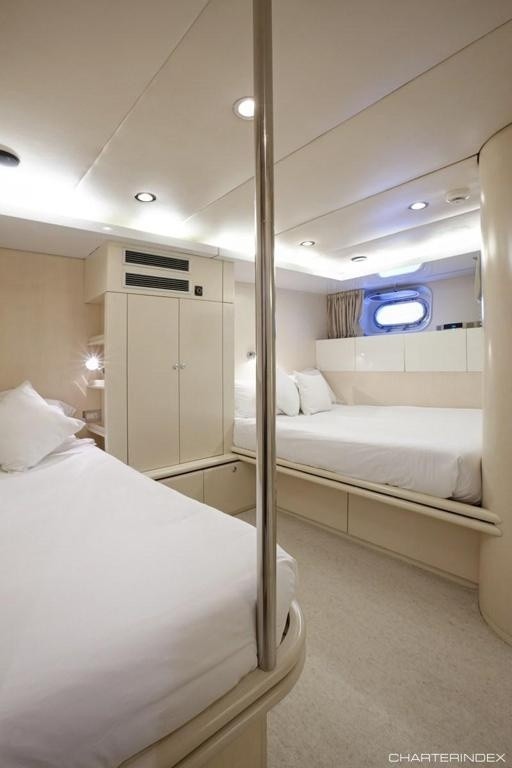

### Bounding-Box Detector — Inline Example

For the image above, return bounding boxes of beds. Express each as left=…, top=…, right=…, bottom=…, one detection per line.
left=233, top=402, right=482, bottom=591
left=0, top=385, right=307, bottom=768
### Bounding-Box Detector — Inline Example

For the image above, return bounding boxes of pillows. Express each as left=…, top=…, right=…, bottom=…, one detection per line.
left=293, top=370, right=332, bottom=416
left=43, top=397, right=79, bottom=455
left=275, top=373, right=301, bottom=417
left=234, top=358, right=257, bottom=417
left=0, top=378, right=84, bottom=472
left=306, top=368, right=337, bottom=403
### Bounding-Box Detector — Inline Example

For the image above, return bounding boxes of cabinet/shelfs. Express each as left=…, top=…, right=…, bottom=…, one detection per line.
left=157, top=460, right=255, bottom=516
left=84, top=240, right=236, bottom=480
